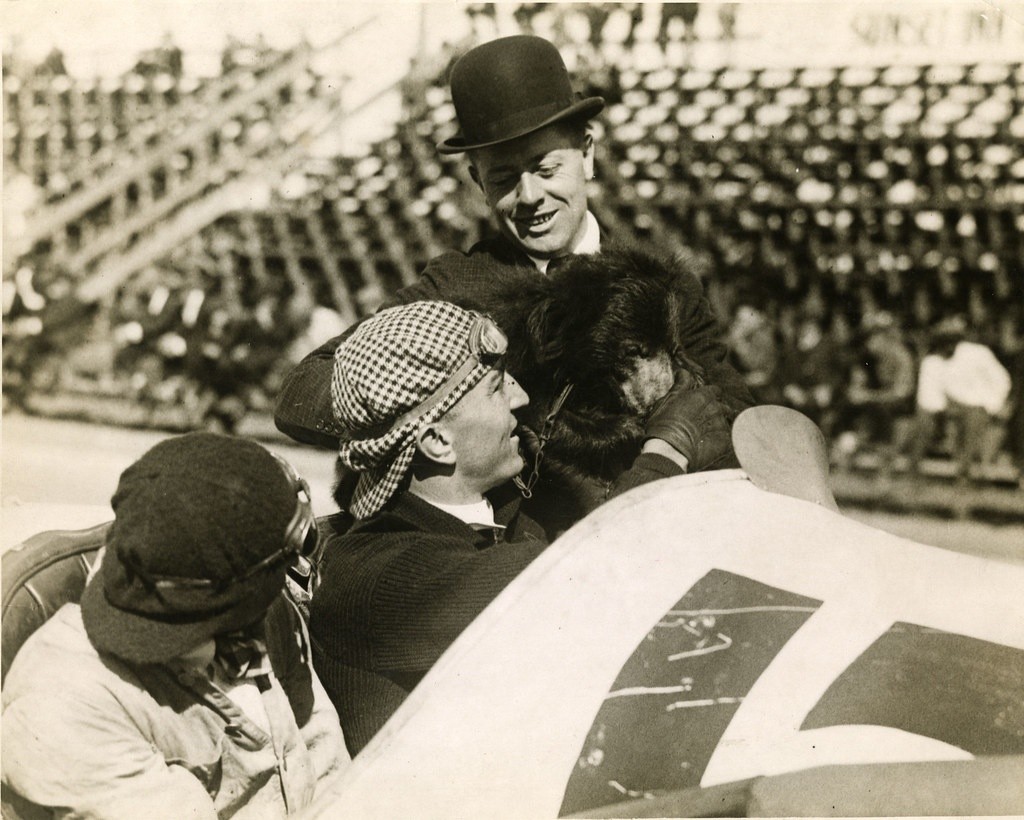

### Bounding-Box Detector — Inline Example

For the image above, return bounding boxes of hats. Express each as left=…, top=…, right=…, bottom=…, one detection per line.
left=329, top=299, right=497, bottom=522
left=434, top=36, right=606, bottom=154
left=81, top=430, right=293, bottom=667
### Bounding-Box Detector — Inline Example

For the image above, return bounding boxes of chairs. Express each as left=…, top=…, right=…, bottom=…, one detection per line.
left=0, top=519, right=113, bottom=820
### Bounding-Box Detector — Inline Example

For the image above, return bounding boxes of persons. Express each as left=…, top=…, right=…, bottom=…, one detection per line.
left=1, top=434, right=353, bottom=820
left=6, top=243, right=81, bottom=403
left=275, top=34, right=752, bottom=526
left=185, top=287, right=391, bottom=442
left=733, top=302, right=1024, bottom=522
left=310, top=302, right=738, bottom=757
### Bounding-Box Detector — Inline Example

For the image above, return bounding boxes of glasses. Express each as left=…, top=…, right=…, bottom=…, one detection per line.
left=468, top=313, right=508, bottom=362
left=238, top=450, right=322, bottom=581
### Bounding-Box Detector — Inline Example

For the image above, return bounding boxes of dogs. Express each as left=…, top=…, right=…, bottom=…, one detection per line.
left=476, top=243, right=691, bottom=509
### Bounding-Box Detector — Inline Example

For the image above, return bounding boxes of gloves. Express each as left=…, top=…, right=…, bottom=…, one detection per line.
left=646, top=372, right=735, bottom=470
